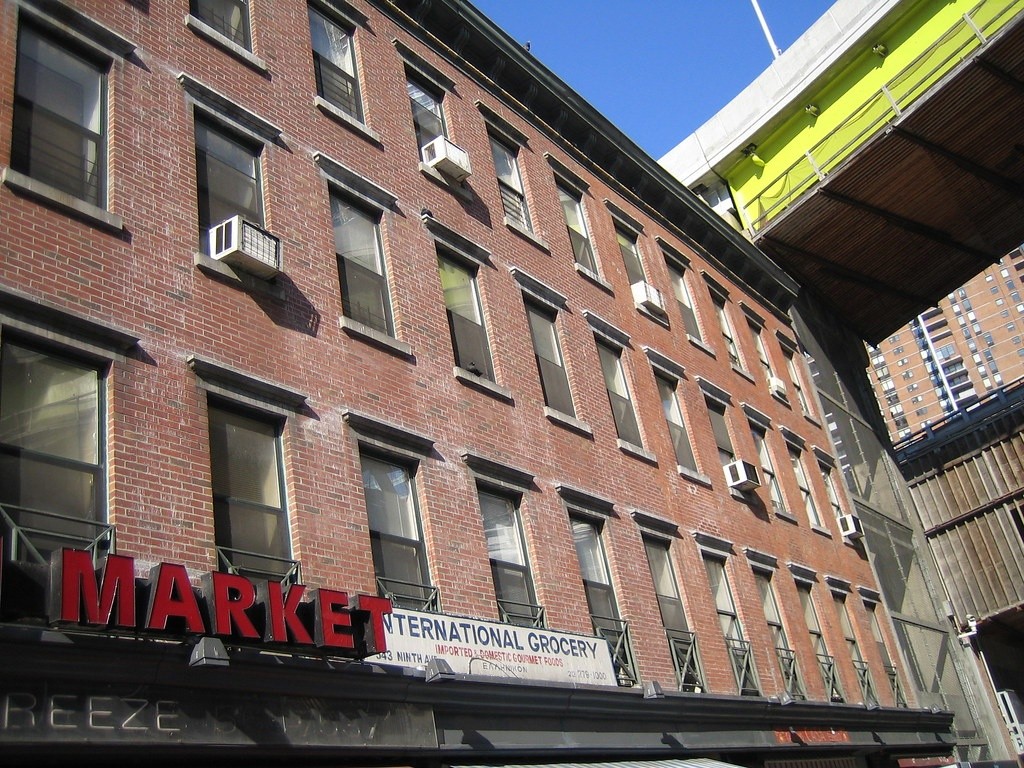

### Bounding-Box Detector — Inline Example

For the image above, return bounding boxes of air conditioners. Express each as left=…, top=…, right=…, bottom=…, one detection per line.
left=836, top=514, right=865, bottom=539
left=768, top=376, right=788, bottom=398
left=419, top=134, right=473, bottom=182
left=630, top=280, right=666, bottom=316
left=208, top=215, right=284, bottom=281
left=723, top=459, right=763, bottom=492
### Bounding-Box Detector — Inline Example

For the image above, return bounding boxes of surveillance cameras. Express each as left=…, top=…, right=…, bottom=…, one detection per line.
left=966, top=614, right=975, bottom=620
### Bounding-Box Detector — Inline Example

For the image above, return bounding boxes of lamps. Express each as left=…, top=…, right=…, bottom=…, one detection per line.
left=929, top=703, right=942, bottom=713
left=863, top=697, right=880, bottom=710
left=770, top=690, right=796, bottom=704
left=184, top=627, right=230, bottom=667
left=631, top=679, right=665, bottom=697
left=419, top=658, right=455, bottom=682
